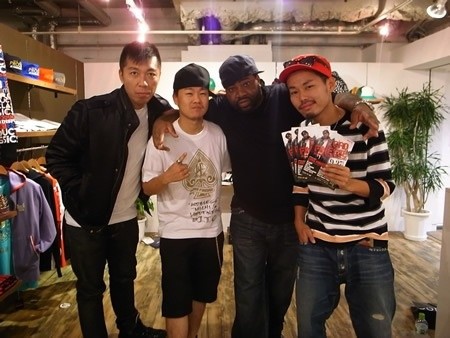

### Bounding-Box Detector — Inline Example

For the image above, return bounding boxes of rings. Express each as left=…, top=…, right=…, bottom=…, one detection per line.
left=177, top=160, right=182, bottom=163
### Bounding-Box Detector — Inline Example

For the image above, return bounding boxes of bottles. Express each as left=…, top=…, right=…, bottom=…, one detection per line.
left=415, top=312, right=428, bottom=335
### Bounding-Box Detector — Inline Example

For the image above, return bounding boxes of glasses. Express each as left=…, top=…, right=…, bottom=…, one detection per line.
left=283, top=56, right=330, bottom=69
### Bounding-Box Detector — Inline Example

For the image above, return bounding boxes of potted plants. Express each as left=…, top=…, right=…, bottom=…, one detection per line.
left=376, top=80, right=450, bottom=242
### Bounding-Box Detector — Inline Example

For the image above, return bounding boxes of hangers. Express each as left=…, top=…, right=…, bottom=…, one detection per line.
left=0, top=145, right=52, bottom=177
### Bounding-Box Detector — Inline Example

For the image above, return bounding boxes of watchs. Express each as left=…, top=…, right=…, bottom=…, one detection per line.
left=352, top=99, right=375, bottom=110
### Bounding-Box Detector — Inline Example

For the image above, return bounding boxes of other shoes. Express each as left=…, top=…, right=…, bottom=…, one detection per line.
left=117, top=322, right=168, bottom=338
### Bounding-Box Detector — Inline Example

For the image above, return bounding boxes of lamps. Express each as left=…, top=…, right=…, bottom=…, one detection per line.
left=426, top=0, right=448, bottom=19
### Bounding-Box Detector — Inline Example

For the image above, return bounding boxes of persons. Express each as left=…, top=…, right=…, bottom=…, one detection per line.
left=284, top=130, right=349, bottom=163
left=142, top=63, right=232, bottom=338
left=153, top=54, right=381, bottom=338
left=45, top=42, right=173, bottom=338
left=278, top=55, right=396, bottom=338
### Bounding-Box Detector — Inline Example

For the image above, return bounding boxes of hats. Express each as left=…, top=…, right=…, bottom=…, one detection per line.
left=3, top=51, right=23, bottom=76
left=219, top=55, right=264, bottom=88
left=52, top=70, right=66, bottom=87
left=174, top=63, right=219, bottom=99
left=278, top=54, right=331, bottom=82
left=37, top=66, right=53, bottom=83
left=21, top=60, right=40, bottom=80
